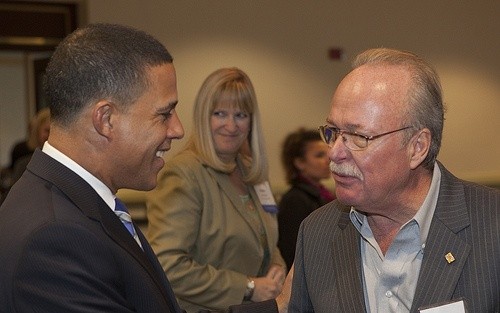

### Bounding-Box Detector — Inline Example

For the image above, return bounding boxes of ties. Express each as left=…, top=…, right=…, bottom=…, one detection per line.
left=113, top=196, right=140, bottom=247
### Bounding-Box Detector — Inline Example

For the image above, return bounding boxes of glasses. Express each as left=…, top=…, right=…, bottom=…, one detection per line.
left=318, top=125, right=413, bottom=151
